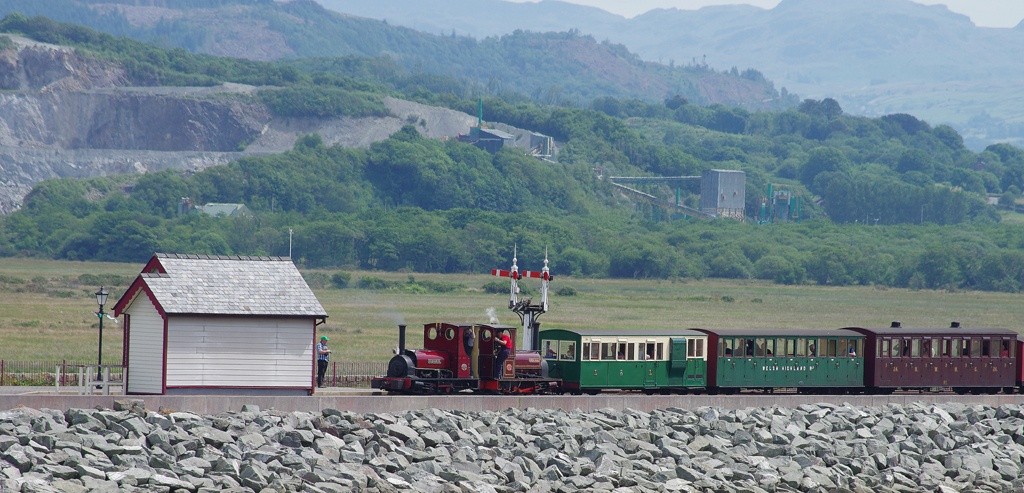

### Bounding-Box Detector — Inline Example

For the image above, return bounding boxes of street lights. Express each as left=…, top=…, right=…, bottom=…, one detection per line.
left=94, top=285, right=111, bottom=388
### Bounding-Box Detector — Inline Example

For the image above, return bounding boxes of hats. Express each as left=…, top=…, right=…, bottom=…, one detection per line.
left=320, top=336, right=330, bottom=341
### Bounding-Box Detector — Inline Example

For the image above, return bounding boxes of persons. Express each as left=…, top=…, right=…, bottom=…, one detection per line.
left=317, top=335, right=332, bottom=388
left=1001, top=344, right=1009, bottom=358
left=809, top=344, right=816, bottom=356
left=893, top=340, right=935, bottom=357
left=464, top=325, right=476, bottom=378
left=638, top=345, right=660, bottom=360
left=544, top=341, right=589, bottom=360
left=723, top=338, right=771, bottom=356
left=493, top=328, right=512, bottom=381
left=611, top=345, right=624, bottom=359
left=842, top=343, right=856, bottom=357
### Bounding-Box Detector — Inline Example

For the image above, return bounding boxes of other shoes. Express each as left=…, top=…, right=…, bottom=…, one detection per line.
left=494, top=376, right=503, bottom=380
left=318, top=386, right=327, bottom=388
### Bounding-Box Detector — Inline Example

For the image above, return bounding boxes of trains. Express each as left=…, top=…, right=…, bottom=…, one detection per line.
left=371, top=315, right=1023, bottom=396
